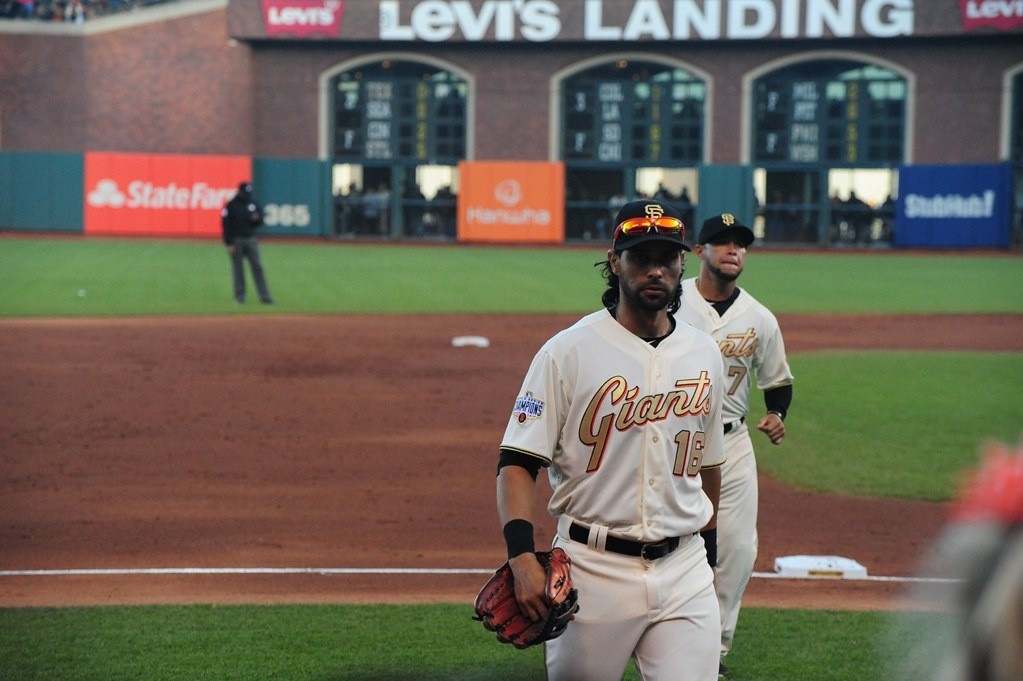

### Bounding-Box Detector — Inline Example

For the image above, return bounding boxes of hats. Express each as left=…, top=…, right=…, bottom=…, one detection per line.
left=240, top=183, right=252, bottom=195
left=697, top=213, right=754, bottom=248
left=611, top=200, right=692, bottom=252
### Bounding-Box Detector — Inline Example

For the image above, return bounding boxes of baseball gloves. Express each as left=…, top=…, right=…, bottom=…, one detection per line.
left=474, top=545, right=580, bottom=649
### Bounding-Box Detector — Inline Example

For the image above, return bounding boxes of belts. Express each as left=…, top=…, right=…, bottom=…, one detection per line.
left=569, top=523, right=699, bottom=563
left=724, top=415, right=745, bottom=434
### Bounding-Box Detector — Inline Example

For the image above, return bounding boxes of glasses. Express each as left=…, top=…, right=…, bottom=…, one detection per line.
left=614, top=217, right=685, bottom=243
left=707, top=232, right=746, bottom=248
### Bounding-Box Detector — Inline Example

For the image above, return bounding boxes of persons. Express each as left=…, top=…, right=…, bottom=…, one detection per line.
left=655, top=182, right=696, bottom=207
left=494, top=198, right=726, bottom=681
left=829, top=191, right=896, bottom=245
left=221, top=183, right=274, bottom=305
left=332, top=180, right=457, bottom=240
left=676, top=214, right=795, bottom=674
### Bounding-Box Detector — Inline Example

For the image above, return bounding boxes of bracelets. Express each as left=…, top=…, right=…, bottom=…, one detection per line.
left=768, top=410, right=783, bottom=421
left=502, top=519, right=535, bottom=562
left=699, top=528, right=717, bottom=568
